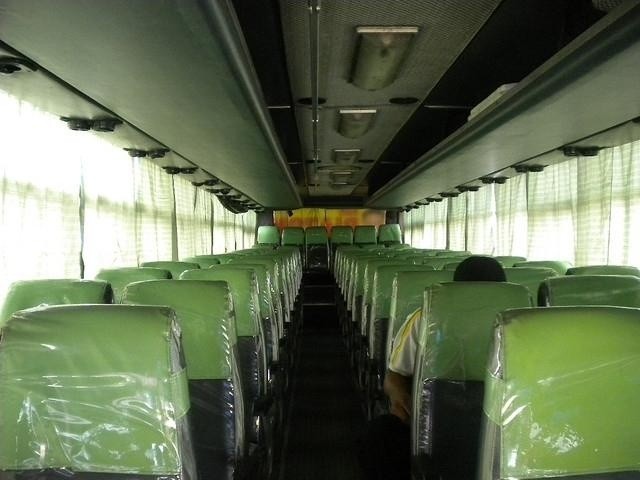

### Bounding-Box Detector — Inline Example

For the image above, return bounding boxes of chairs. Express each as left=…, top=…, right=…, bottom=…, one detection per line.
left=412, top=281, right=533, bottom=479
left=537, top=274, right=640, bottom=309
left=119, top=278, right=260, bottom=479
left=256, top=223, right=402, bottom=273
left=478, top=306, right=640, bottom=480
left=92, top=245, right=305, bottom=479
left=332, top=243, right=566, bottom=435
left=566, top=265, right=638, bottom=277
left=1, top=278, right=114, bottom=324
left=1, top=303, right=199, bottom=480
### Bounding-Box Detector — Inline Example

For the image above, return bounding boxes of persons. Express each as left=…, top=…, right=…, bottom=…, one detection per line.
left=366, top=256, right=508, bottom=480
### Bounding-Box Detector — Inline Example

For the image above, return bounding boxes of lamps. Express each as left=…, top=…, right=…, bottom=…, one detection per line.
left=328, top=25, right=418, bottom=189
